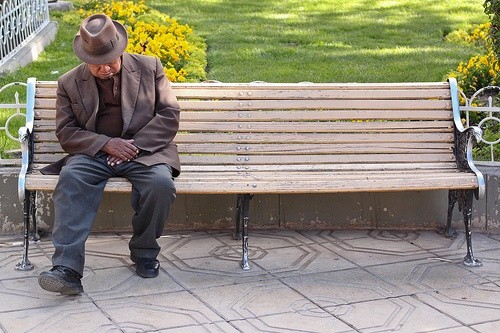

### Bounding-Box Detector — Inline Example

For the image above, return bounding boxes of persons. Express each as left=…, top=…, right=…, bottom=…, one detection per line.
left=38, top=15, right=181, bottom=297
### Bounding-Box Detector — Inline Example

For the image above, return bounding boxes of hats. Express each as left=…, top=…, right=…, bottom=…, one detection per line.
left=73, top=14, right=128, bottom=65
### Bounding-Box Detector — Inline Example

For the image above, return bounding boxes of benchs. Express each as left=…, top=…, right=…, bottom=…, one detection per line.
left=13, top=76, right=486, bottom=271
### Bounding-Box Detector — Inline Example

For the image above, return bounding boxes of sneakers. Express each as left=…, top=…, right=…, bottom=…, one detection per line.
left=38, top=266, right=83, bottom=295
left=130, top=254, right=160, bottom=278
